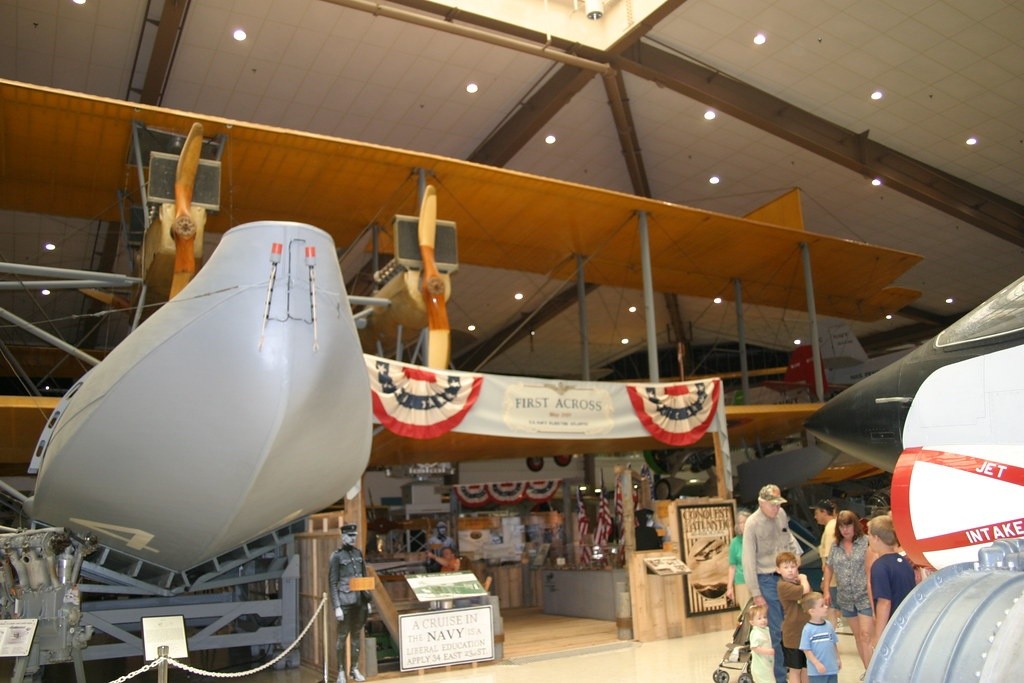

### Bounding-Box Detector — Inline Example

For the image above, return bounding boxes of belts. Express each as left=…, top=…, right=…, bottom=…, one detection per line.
left=770, top=572, right=781, bottom=577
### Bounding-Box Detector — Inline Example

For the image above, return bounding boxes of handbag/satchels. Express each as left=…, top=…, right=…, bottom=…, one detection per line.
left=361, top=591, right=372, bottom=604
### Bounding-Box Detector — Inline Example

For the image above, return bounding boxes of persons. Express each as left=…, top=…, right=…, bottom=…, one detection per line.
left=425, top=547, right=461, bottom=572
left=328, top=524, right=372, bottom=683
left=430, top=521, right=455, bottom=556
left=635, top=477, right=921, bottom=683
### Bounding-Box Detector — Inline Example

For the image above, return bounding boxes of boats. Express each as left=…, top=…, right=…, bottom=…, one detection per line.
left=13, top=219, right=371, bottom=576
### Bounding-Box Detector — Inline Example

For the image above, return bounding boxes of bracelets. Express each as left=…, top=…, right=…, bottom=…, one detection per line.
left=431, top=555, right=436, bottom=560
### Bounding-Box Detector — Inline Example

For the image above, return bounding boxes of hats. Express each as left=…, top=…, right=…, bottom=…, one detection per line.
left=341, top=524, right=358, bottom=535
left=808, top=499, right=834, bottom=511
left=757, top=484, right=788, bottom=503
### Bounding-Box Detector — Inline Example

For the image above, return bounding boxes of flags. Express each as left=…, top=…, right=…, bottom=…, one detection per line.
left=577, top=468, right=638, bottom=570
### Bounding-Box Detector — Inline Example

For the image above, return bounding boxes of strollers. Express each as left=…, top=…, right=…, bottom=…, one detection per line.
left=712, top=595, right=756, bottom=683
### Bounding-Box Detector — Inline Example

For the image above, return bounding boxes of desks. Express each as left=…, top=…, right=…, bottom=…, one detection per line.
left=541, top=568, right=628, bottom=623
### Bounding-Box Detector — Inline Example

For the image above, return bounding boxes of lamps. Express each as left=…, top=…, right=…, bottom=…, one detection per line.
left=591, top=543, right=619, bottom=561
